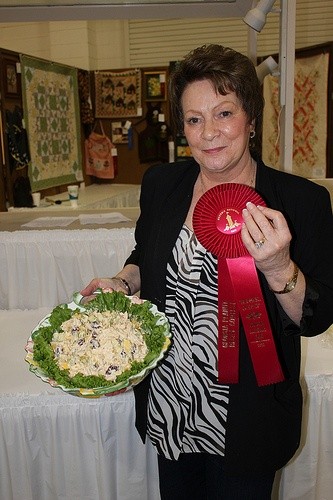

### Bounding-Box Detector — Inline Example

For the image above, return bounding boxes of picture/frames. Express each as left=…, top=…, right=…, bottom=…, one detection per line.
left=3, top=56, right=21, bottom=99
left=144, top=71, right=168, bottom=102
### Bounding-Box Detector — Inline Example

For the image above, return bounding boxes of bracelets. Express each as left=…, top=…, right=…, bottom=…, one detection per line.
left=112, top=276, right=131, bottom=296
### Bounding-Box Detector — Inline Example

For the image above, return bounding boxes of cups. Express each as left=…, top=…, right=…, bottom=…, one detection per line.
left=68, top=186, right=78, bottom=200
left=32, top=193, right=40, bottom=207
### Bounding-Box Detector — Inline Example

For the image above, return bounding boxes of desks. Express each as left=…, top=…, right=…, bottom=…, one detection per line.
left=30, top=182, right=141, bottom=211
left=0, top=210, right=142, bottom=308
left=0, top=310, right=333, bottom=500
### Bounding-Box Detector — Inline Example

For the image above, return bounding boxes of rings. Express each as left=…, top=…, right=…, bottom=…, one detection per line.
left=255, top=237, right=265, bottom=248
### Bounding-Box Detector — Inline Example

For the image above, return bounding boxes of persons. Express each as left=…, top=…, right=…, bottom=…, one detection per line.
left=80, top=44, right=333, bottom=500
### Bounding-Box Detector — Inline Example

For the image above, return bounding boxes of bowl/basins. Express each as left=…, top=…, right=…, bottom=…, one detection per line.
left=24, top=289, right=173, bottom=399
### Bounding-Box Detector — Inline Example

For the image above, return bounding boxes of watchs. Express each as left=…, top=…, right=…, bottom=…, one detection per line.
left=271, top=262, right=298, bottom=294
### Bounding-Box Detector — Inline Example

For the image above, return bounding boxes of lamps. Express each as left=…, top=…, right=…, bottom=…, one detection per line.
left=243, top=0, right=281, bottom=33
left=255, top=55, right=280, bottom=89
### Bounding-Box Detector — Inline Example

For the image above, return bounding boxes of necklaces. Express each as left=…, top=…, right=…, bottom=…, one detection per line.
left=200, top=164, right=255, bottom=193
